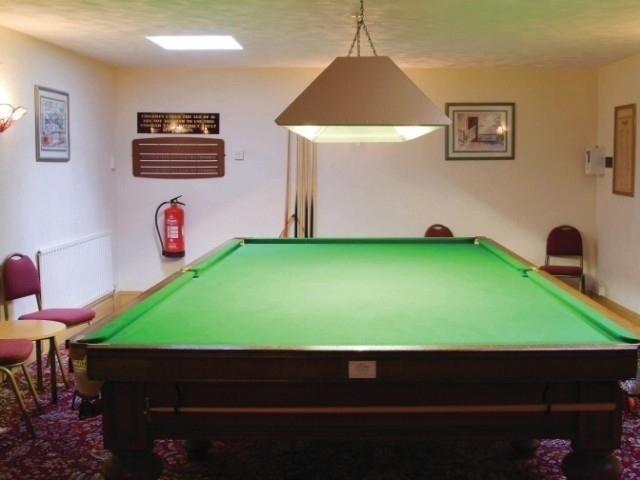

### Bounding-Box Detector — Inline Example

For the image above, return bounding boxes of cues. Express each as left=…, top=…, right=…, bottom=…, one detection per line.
left=283, top=126, right=316, bottom=239
left=150, top=403, right=617, bottom=412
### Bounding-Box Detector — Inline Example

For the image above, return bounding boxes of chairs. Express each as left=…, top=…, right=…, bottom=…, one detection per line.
left=0, top=253, right=97, bottom=438
left=424, top=224, right=452, bottom=237
left=538, top=225, right=585, bottom=296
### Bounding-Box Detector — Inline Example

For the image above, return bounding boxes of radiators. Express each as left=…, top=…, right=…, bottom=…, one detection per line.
left=35, top=231, right=119, bottom=314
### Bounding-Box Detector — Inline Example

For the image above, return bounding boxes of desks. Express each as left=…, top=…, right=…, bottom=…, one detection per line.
left=66, top=239, right=640, bottom=480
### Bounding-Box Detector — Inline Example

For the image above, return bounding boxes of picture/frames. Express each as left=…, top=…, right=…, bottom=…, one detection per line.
left=612, top=104, right=635, bottom=196
left=444, top=102, right=515, bottom=162
left=34, top=83, right=71, bottom=162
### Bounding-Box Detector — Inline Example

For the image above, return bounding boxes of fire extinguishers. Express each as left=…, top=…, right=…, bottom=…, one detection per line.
left=155, top=195, right=186, bottom=257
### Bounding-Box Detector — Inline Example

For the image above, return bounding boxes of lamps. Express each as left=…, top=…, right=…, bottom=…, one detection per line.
left=0, top=88, right=27, bottom=132
left=274, top=0, right=451, bottom=145
